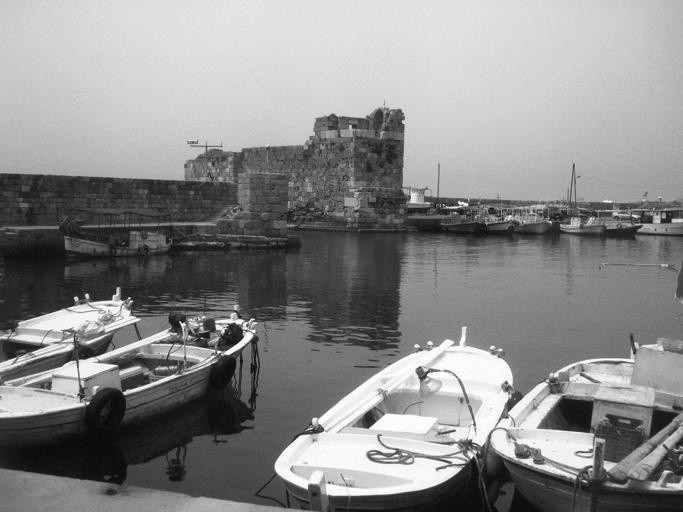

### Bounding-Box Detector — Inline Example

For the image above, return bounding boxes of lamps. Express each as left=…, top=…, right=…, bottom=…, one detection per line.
left=416, top=363, right=482, bottom=440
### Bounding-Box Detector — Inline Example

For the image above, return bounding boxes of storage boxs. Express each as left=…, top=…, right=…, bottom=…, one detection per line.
left=590, top=380, right=657, bottom=443
left=50, top=360, right=122, bottom=399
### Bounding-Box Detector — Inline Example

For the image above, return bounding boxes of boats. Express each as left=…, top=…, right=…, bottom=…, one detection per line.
left=0, top=304, right=257, bottom=452
left=273, top=326, right=513, bottom=511
left=62, top=204, right=172, bottom=258
left=405, top=161, right=682, bottom=236
left=489, top=334, right=683, bottom=511
left=0, top=286, right=141, bottom=386
